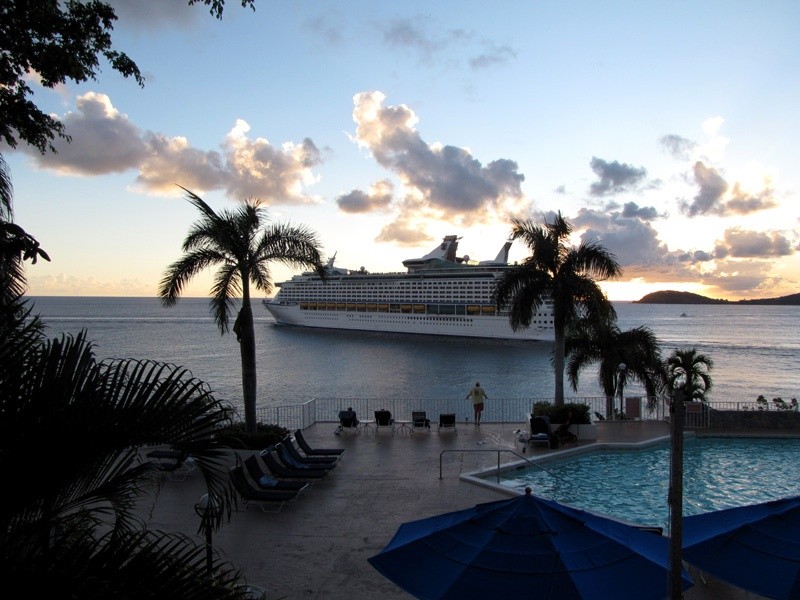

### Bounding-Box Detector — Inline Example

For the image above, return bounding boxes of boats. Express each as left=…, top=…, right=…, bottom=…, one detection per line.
left=413, top=305, right=425, bottom=311
left=337, top=304, right=345, bottom=308
left=327, top=304, right=336, bottom=308
left=301, top=303, right=308, bottom=307
left=368, top=304, right=377, bottom=309
left=318, top=303, right=326, bottom=307
left=357, top=304, right=366, bottom=309
left=482, top=306, right=495, bottom=312
left=347, top=303, right=355, bottom=309
left=379, top=304, right=389, bottom=309
left=400, top=304, right=412, bottom=310
left=310, top=303, right=317, bottom=307
left=467, top=305, right=480, bottom=312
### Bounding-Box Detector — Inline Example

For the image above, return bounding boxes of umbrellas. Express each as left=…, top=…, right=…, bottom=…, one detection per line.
left=367, top=487, right=695, bottom=599
left=678, top=495, right=800, bottom=600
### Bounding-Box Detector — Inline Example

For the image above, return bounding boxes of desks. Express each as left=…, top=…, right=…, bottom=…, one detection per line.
left=395, top=419, right=412, bottom=437
left=513, top=429, right=528, bottom=448
left=359, top=419, right=376, bottom=433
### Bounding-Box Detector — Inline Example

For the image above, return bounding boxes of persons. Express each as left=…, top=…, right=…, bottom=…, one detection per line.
left=256, top=470, right=305, bottom=489
left=466, top=382, right=488, bottom=426
left=348, top=407, right=352, bottom=411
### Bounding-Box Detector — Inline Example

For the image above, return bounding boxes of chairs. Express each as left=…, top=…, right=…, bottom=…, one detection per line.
left=339, top=408, right=359, bottom=436
left=375, top=409, right=395, bottom=437
left=243, top=453, right=316, bottom=495
left=294, top=427, right=346, bottom=459
left=146, top=444, right=200, bottom=482
left=436, top=412, right=458, bottom=437
left=283, top=432, right=339, bottom=465
left=529, top=414, right=551, bottom=449
left=411, top=409, right=431, bottom=438
left=259, top=443, right=329, bottom=487
left=229, top=463, right=309, bottom=515
left=275, top=439, right=338, bottom=472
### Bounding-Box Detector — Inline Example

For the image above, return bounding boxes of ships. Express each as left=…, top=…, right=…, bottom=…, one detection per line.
left=262, top=232, right=588, bottom=342
